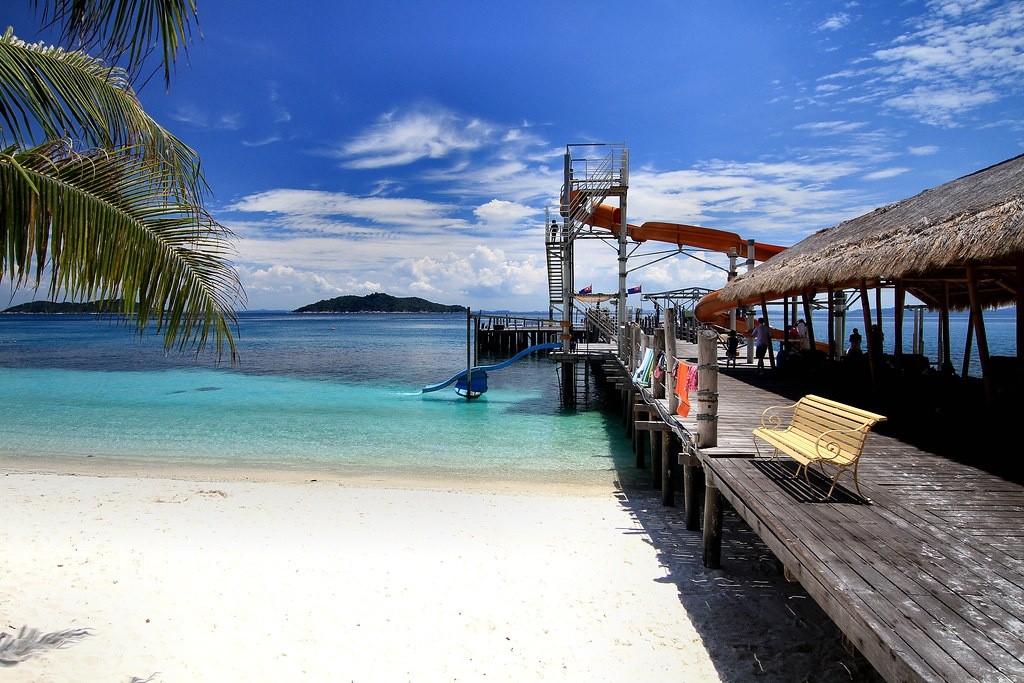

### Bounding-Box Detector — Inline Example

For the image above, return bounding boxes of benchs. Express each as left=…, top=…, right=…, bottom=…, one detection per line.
left=751, top=394, right=888, bottom=503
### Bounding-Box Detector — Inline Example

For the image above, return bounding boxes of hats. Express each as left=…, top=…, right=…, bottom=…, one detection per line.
left=757, top=318, right=766, bottom=323
left=728, top=330, right=736, bottom=336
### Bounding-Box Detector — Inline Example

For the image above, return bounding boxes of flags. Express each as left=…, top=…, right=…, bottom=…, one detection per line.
left=579, top=286, right=591, bottom=294
left=627, top=286, right=641, bottom=294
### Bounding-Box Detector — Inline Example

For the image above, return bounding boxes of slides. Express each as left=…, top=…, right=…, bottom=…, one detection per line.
left=559, top=190, right=829, bottom=351
left=421, top=342, right=563, bottom=393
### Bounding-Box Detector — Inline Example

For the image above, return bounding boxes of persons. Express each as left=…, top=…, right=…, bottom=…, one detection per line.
left=849, top=328, right=861, bottom=349
left=870, top=324, right=885, bottom=342
left=788, top=319, right=806, bottom=338
left=726, top=330, right=738, bottom=368
left=550, top=220, right=559, bottom=241
left=750, top=318, right=773, bottom=373
